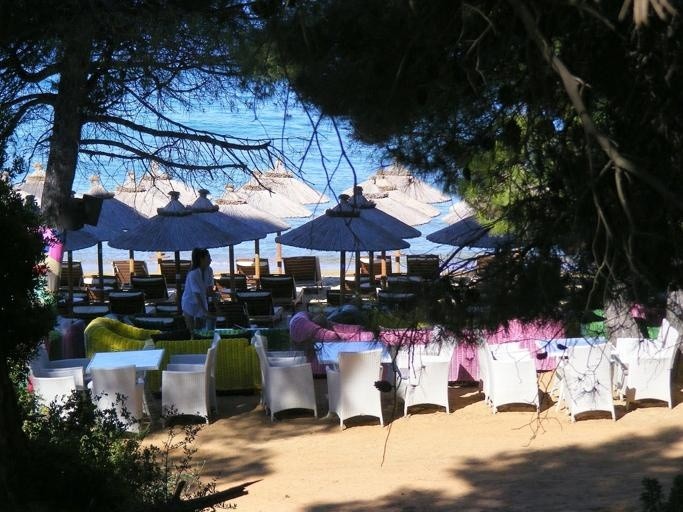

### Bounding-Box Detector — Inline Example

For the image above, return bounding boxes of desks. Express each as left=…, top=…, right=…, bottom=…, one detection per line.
left=85, top=348, right=165, bottom=412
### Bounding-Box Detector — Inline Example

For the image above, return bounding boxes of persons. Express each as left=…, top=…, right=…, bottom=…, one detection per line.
left=181, top=249, right=216, bottom=339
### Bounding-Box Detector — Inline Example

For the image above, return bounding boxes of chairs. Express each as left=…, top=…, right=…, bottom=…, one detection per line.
left=246, top=330, right=320, bottom=426
left=160, top=332, right=222, bottom=427
left=55, top=253, right=499, bottom=340
left=81, top=314, right=270, bottom=390
left=396, top=324, right=459, bottom=420
left=474, top=318, right=681, bottom=424
left=86, top=363, right=147, bottom=428
left=322, top=347, right=387, bottom=430
left=23, top=342, right=91, bottom=418
